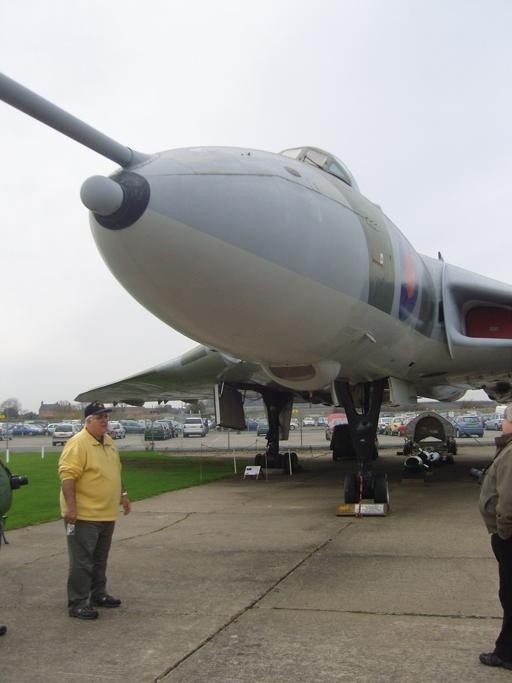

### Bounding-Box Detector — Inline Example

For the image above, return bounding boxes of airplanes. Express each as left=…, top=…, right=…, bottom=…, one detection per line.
left=0, top=71, right=511, bottom=503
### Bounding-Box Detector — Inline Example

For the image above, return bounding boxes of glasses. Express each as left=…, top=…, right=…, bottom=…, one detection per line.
left=499, top=414, right=508, bottom=422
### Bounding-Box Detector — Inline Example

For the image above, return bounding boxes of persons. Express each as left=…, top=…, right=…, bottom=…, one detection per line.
left=56, top=399, right=132, bottom=620
left=476, top=403, right=512, bottom=674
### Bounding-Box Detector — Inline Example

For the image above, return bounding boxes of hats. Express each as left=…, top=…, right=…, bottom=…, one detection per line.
left=85, top=400, right=113, bottom=418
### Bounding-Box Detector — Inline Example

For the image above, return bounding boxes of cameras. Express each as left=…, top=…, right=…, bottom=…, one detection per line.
left=66, top=523, right=75, bottom=536
left=470, top=468, right=487, bottom=485
left=10, top=475, right=28, bottom=489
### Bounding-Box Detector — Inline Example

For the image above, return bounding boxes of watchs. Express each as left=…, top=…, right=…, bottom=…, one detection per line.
left=122, top=488, right=128, bottom=497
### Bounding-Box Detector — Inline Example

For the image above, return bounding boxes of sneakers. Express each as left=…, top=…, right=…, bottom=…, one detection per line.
left=479, top=652, right=512, bottom=670
left=69, top=606, right=99, bottom=620
left=90, top=594, right=121, bottom=609
